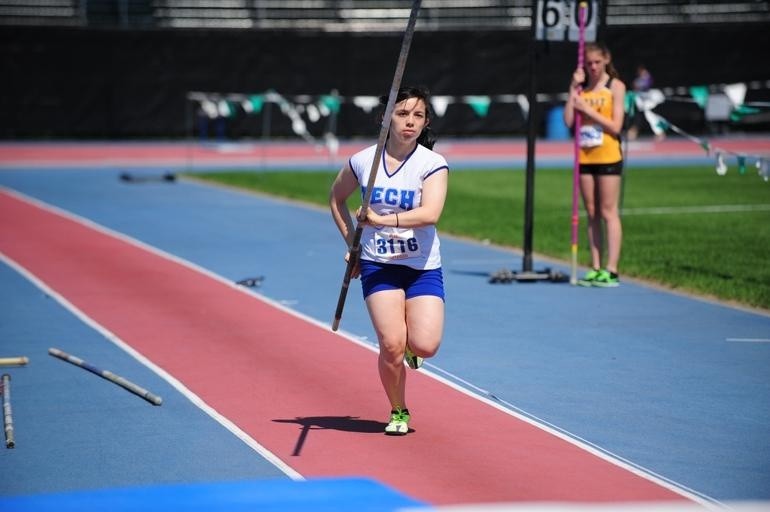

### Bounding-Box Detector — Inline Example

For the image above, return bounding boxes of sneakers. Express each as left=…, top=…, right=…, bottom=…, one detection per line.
left=385, top=406, right=410, bottom=435
left=590, top=269, right=620, bottom=288
left=404, top=344, right=424, bottom=369
left=578, top=269, right=600, bottom=288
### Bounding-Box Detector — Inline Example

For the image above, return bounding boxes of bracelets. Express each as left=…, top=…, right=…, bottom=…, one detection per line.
left=396, top=212, right=399, bottom=227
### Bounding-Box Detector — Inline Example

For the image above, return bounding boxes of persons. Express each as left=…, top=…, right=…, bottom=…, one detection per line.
left=563, top=46, right=626, bottom=288
left=329, top=85, right=449, bottom=436
left=627, top=64, right=666, bottom=142
